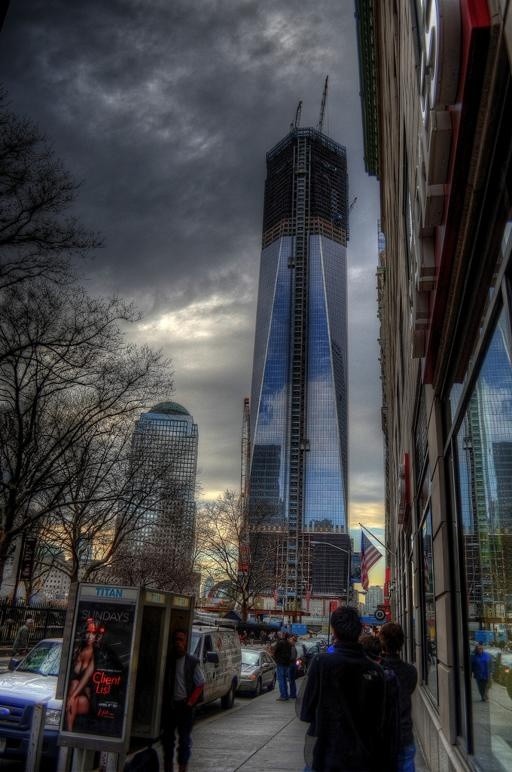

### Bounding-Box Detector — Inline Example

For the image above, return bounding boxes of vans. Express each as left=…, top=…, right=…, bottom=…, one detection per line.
left=186, top=625, right=242, bottom=712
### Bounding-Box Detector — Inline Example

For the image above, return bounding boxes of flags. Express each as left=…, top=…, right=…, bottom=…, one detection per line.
left=359, top=530, right=383, bottom=592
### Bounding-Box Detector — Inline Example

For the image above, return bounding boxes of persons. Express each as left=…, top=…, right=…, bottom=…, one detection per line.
left=426, top=633, right=434, bottom=675
left=8, top=618, right=35, bottom=671
left=238, top=606, right=419, bottom=772
left=471, top=643, right=492, bottom=702
left=159, top=627, right=208, bottom=771
left=65, top=618, right=102, bottom=734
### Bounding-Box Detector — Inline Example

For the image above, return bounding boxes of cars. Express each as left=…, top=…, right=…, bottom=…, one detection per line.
left=235, top=646, right=277, bottom=696
left=0, top=634, right=63, bottom=771
left=292, top=635, right=333, bottom=677
